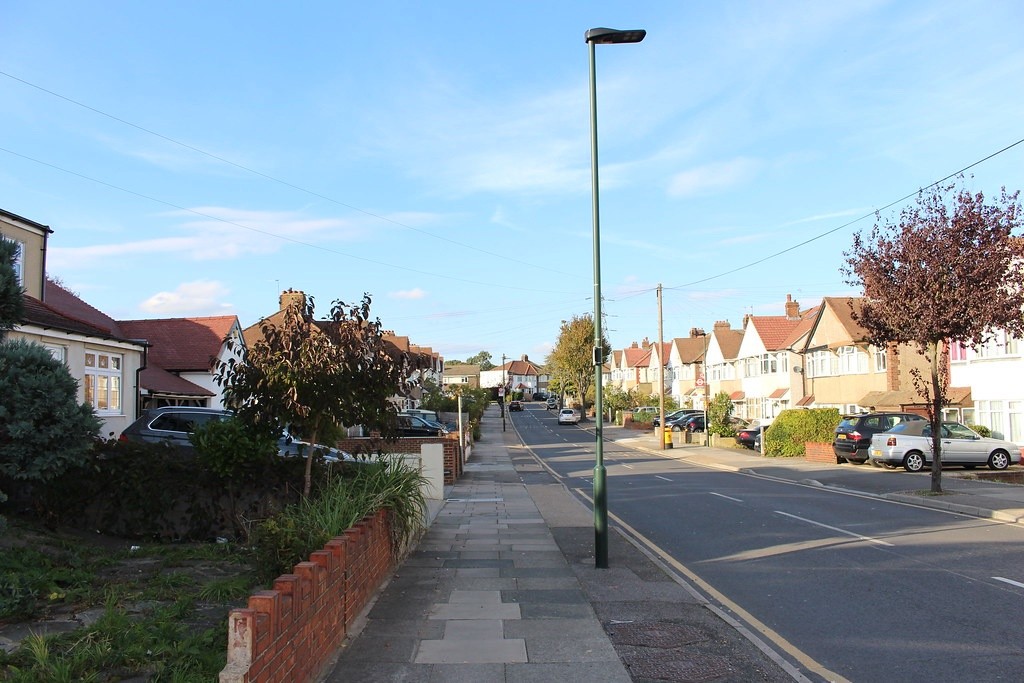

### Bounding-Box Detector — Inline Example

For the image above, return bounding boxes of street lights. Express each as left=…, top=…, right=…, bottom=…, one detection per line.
left=693, top=333, right=709, bottom=446
left=585, top=27, right=647, bottom=566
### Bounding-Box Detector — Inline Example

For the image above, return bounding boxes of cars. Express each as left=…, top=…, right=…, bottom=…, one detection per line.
left=363, top=416, right=444, bottom=437
left=558, top=409, right=578, bottom=425
left=686, top=415, right=710, bottom=433
left=533, top=393, right=549, bottom=401
left=652, top=409, right=704, bottom=427
left=665, top=413, right=704, bottom=432
left=868, top=420, right=1021, bottom=472
left=546, top=399, right=557, bottom=410
left=509, top=401, right=524, bottom=412
left=118, top=406, right=356, bottom=461
left=734, top=425, right=770, bottom=450
left=754, top=435, right=761, bottom=453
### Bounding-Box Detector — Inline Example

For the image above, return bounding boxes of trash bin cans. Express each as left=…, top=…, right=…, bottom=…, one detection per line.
left=665, top=427, right=674, bottom=450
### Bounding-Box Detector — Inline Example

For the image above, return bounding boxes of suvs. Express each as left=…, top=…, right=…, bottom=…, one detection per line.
left=832, top=411, right=929, bottom=468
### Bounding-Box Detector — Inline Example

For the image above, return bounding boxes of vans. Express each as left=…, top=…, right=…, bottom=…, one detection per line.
left=633, top=407, right=670, bottom=414
